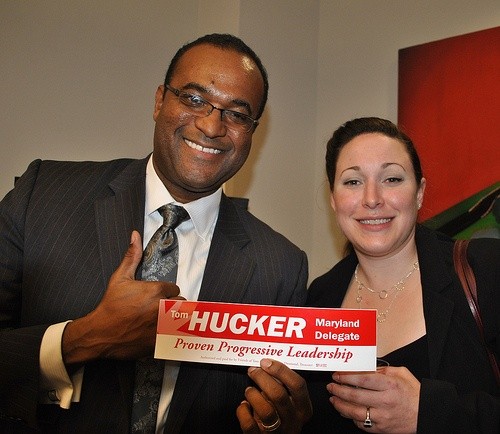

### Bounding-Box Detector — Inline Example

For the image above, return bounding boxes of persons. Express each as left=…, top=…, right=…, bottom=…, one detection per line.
left=297, top=116, right=499, bottom=434
left=0, top=33, right=318, bottom=434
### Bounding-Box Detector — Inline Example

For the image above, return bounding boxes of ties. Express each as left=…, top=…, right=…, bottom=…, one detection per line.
left=131, top=204, right=191, bottom=434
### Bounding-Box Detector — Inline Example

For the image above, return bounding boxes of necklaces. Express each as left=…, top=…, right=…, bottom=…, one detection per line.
left=354, top=256, right=420, bottom=323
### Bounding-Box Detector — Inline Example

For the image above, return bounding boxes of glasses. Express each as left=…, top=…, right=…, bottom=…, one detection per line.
left=167, top=84, right=259, bottom=132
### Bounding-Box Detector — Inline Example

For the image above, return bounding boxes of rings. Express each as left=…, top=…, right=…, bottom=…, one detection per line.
left=363, top=407, right=372, bottom=428
left=261, top=408, right=282, bottom=432
